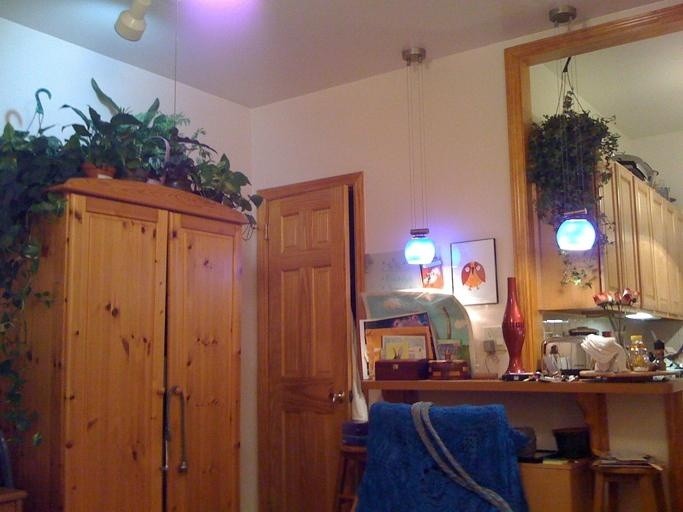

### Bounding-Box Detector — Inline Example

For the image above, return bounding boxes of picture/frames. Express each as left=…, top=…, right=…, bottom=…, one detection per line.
left=436, top=339, right=461, bottom=360
left=450, top=238, right=498, bottom=306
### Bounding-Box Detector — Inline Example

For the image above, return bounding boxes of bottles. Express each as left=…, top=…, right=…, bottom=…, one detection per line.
left=653, top=339, right=666, bottom=371
left=629, top=334, right=648, bottom=372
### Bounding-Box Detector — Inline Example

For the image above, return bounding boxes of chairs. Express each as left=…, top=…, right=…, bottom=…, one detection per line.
left=355, top=400, right=532, bottom=512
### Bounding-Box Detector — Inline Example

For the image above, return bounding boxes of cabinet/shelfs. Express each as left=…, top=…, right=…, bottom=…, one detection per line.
left=532, top=161, right=683, bottom=321
left=0, top=178, right=250, bottom=512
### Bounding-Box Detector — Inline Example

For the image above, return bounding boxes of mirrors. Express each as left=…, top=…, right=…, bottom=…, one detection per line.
left=504, top=3, right=683, bottom=373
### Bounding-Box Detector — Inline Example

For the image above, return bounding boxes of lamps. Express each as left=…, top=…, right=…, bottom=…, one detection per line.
left=549, top=5, right=596, bottom=251
left=402, top=47, right=436, bottom=264
left=115, top=0, right=152, bottom=41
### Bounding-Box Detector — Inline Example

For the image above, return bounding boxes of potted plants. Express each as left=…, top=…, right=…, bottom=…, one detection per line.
left=526, top=57, right=622, bottom=289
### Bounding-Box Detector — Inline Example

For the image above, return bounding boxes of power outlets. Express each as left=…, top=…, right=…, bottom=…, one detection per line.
left=483, top=325, right=507, bottom=351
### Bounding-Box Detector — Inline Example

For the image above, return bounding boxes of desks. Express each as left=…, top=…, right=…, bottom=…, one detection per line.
left=361, top=380, right=683, bottom=512
left=0, top=487, right=27, bottom=512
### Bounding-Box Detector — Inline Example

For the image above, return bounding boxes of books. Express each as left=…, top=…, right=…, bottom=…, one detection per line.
left=380, top=332, right=427, bottom=365
left=357, top=312, right=439, bottom=380
left=365, top=328, right=434, bottom=377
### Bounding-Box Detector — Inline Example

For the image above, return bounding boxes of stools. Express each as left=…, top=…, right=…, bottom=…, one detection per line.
left=592, top=460, right=667, bottom=512
left=332, top=445, right=367, bottom=512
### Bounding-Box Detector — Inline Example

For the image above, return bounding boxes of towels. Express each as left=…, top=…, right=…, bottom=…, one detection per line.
left=580, top=334, right=629, bottom=372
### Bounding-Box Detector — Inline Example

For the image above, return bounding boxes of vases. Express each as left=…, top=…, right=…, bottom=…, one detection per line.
left=502, top=277, right=526, bottom=375
left=615, top=331, right=630, bottom=369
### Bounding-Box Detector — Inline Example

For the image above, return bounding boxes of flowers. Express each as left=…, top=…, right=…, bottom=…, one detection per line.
left=593, top=288, right=640, bottom=348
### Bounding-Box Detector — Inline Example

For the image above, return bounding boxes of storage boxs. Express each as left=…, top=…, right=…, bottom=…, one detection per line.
left=521, top=459, right=592, bottom=512
